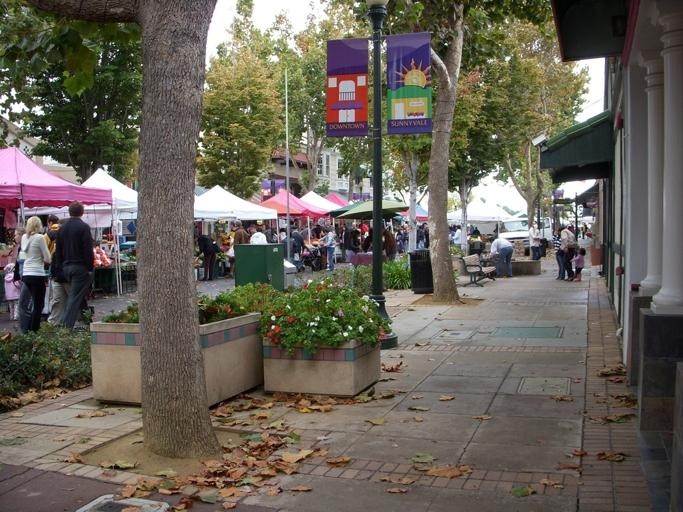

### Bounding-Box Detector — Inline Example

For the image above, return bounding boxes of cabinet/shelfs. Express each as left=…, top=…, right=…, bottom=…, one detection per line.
left=91, top=259, right=137, bottom=298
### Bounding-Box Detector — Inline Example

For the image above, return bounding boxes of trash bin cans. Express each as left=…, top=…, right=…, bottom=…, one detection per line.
left=407, top=248, right=434, bottom=294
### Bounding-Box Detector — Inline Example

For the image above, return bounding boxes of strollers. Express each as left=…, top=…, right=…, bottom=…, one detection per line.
left=293, top=241, right=322, bottom=275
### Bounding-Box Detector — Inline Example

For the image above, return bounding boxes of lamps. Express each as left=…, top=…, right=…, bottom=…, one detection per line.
left=531, top=134, right=546, bottom=150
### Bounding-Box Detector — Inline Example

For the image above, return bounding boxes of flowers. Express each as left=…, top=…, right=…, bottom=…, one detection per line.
left=89, top=295, right=263, bottom=325
left=256, top=277, right=392, bottom=359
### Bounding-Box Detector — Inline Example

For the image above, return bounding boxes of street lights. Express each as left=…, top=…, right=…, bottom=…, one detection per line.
left=362, top=0, right=400, bottom=349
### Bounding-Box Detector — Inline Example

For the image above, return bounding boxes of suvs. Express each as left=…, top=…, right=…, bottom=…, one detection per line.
left=500, top=217, right=553, bottom=252
left=458, top=216, right=506, bottom=255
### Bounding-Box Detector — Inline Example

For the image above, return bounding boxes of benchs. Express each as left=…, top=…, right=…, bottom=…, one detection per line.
left=460, top=253, right=497, bottom=288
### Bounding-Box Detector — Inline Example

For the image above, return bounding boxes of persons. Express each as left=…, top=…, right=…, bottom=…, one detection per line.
left=487, top=237, right=513, bottom=278
left=0, top=201, right=93, bottom=333
left=550, top=222, right=592, bottom=282
left=194, top=218, right=483, bottom=282
left=528, top=220, right=541, bottom=260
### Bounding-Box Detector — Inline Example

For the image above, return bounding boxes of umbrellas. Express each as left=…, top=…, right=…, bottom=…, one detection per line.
left=328, top=199, right=400, bottom=218
left=335, top=200, right=408, bottom=217
